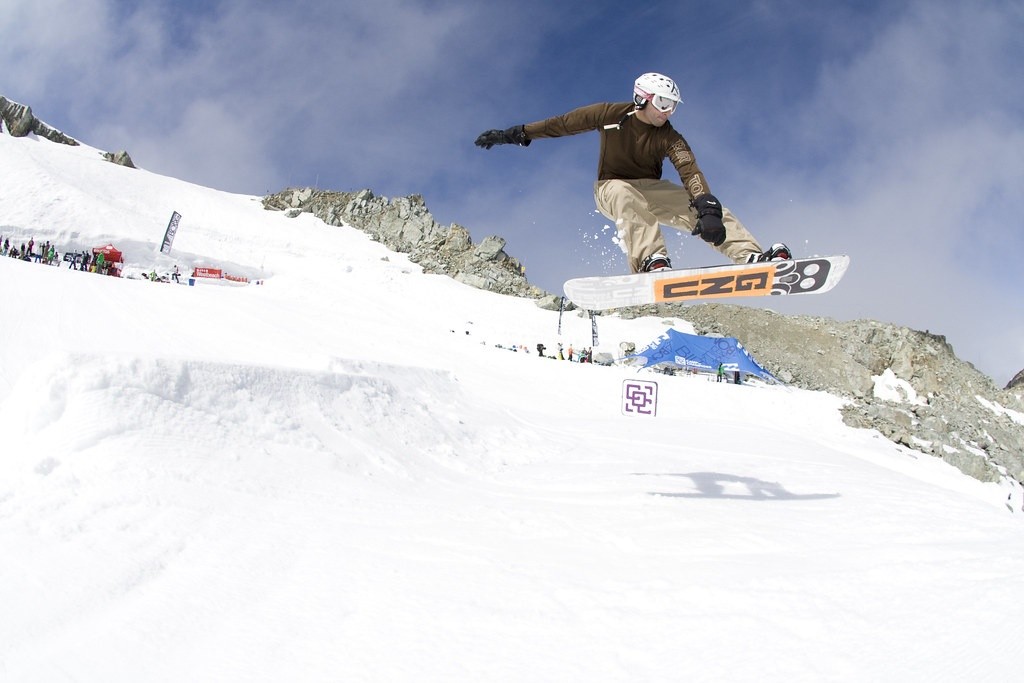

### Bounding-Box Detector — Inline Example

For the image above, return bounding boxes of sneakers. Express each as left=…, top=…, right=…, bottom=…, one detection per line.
left=641, top=253, right=672, bottom=272
left=759, top=242, right=792, bottom=262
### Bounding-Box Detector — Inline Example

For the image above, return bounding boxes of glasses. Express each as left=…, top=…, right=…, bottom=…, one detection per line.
left=652, top=94, right=678, bottom=114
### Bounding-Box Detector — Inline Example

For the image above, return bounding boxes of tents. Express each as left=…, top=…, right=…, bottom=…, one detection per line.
left=92, top=243, right=124, bottom=276
left=602, top=329, right=785, bottom=386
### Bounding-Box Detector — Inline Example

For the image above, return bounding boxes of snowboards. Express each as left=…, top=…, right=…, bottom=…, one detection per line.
left=563, top=253, right=851, bottom=312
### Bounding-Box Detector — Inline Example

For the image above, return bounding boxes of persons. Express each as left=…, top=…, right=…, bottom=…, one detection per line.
left=0, top=236, right=182, bottom=283
left=716, top=361, right=724, bottom=383
left=664, top=366, right=668, bottom=374
left=534, top=341, right=594, bottom=364
left=475, top=73, right=793, bottom=273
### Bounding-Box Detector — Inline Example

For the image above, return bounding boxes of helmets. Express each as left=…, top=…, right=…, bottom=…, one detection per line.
left=633, top=72, right=684, bottom=109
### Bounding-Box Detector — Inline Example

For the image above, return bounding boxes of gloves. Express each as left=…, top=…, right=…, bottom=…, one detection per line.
left=474, top=124, right=532, bottom=150
left=688, top=193, right=726, bottom=246
